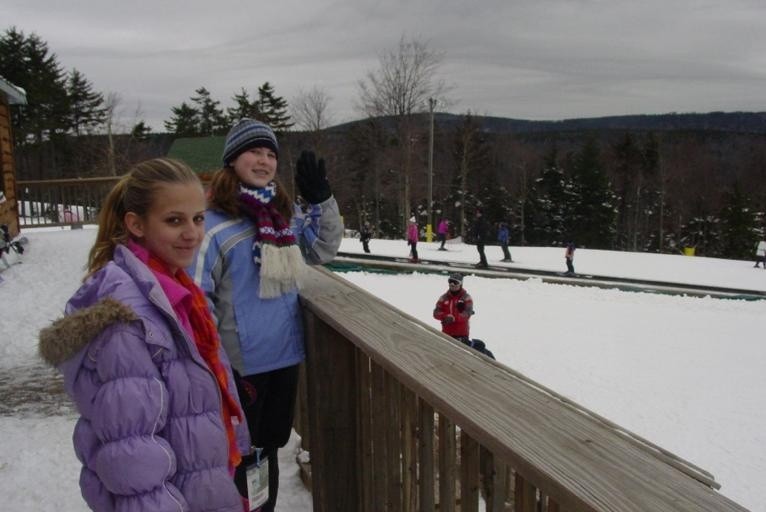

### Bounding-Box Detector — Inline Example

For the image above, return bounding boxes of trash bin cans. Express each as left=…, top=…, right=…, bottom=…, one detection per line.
left=684, top=246, right=695, bottom=256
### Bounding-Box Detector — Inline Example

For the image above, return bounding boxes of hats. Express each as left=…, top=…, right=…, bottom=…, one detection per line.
left=223, top=118, right=278, bottom=162
left=450, top=273, right=463, bottom=284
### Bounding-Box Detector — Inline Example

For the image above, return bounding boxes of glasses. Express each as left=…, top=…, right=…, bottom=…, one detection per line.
left=448, top=279, right=459, bottom=285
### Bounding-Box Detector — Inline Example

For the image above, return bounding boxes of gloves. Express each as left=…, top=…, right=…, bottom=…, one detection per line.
left=295, top=150, right=331, bottom=204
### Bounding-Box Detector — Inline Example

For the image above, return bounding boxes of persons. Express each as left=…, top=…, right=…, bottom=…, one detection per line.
left=453, top=334, right=509, bottom=511
left=181, top=118, right=345, bottom=512
left=753, top=235, right=766, bottom=269
left=406, top=215, right=419, bottom=263
left=432, top=272, right=472, bottom=341
left=39, top=157, right=254, bottom=512
left=466, top=212, right=489, bottom=268
left=564, top=240, right=576, bottom=274
left=359, top=221, right=371, bottom=253
left=496, top=221, right=512, bottom=263
left=437, top=217, right=450, bottom=251
